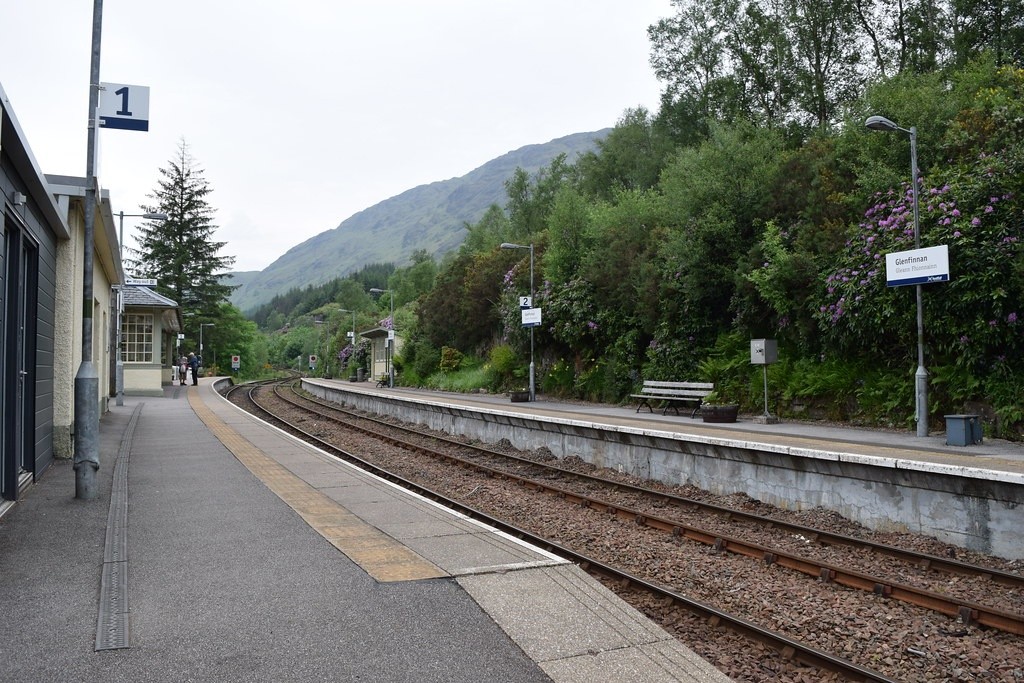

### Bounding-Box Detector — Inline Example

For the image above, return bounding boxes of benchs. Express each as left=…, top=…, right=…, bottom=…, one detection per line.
left=629, top=380, right=714, bottom=419
left=375, top=371, right=390, bottom=388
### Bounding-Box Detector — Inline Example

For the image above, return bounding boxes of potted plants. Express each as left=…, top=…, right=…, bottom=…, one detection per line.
left=700, top=390, right=740, bottom=423
left=509, top=384, right=530, bottom=402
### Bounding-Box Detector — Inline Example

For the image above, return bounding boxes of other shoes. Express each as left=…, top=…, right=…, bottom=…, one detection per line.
left=191, top=384, right=197, bottom=386
left=180, top=383, right=186, bottom=386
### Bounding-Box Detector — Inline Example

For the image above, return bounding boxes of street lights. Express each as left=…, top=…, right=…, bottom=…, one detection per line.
left=199, top=323, right=215, bottom=367
left=501, top=242, right=536, bottom=403
left=337, top=309, right=356, bottom=376
left=113, top=210, right=169, bottom=264
left=315, top=320, right=330, bottom=378
left=865, top=114, right=930, bottom=437
left=370, top=288, right=395, bottom=388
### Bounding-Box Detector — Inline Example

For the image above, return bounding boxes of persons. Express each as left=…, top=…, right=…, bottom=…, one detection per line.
left=177, top=352, right=188, bottom=386
left=188, top=352, right=199, bottom=386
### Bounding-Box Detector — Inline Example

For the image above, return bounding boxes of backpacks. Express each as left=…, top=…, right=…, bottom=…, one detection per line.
left=180, top=364, right=186, bottom=373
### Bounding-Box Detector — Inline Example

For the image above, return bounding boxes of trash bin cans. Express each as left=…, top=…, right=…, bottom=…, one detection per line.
left=357, top=367, right=364, bottom=381
